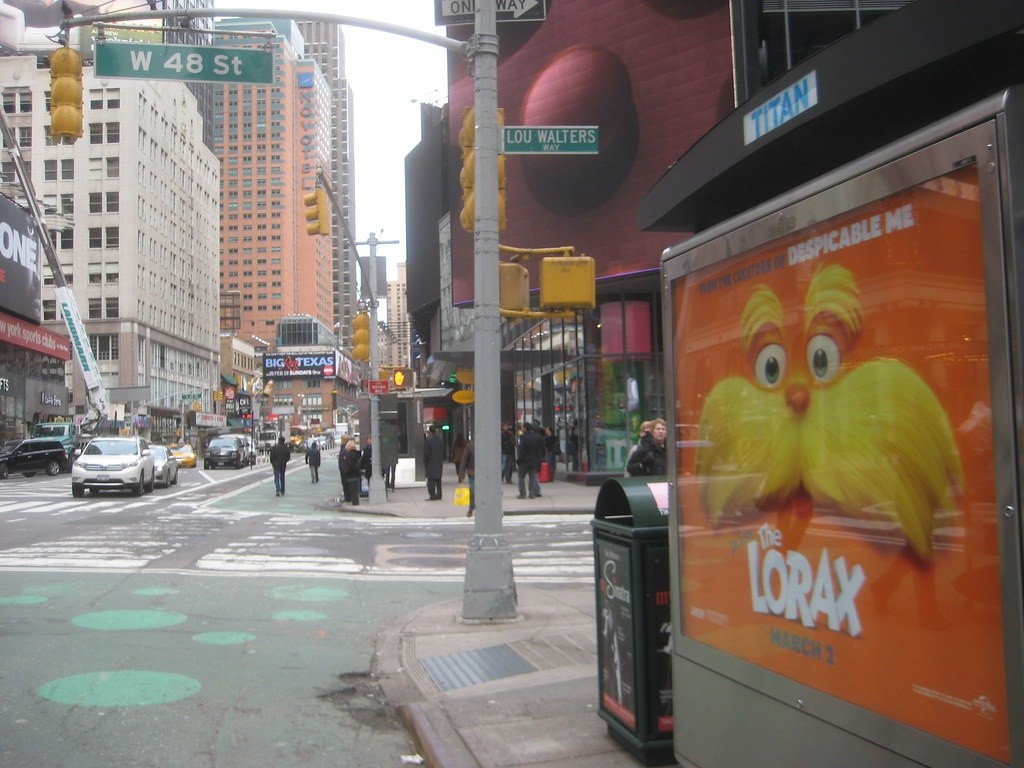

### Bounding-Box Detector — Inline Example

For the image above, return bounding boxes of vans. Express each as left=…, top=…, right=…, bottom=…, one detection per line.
left=256, top=430, right=280, bottom=451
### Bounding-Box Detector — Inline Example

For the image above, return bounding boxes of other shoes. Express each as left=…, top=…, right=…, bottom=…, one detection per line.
left=529, top=495, right=534, bottom=498
left=316, top=479, right=319, bottom=482
left=466, top=510, right=472, bottom=518
left=281, top=491, right=285, bottom=495
left=517, top=495, right=526, bottom=499
left=535, top=493, right=542, bottom=497
left=506, top=481, right=513, bottom=484
left=312, top=481, right=314, bottom=483
left=276, top=493, right=279, bottom=496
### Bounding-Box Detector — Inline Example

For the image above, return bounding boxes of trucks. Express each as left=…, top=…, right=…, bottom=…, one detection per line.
left=31, top=422, right=99, bottom=472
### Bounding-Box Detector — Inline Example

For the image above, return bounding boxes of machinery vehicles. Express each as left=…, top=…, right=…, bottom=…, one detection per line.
left=0, top=100, right=134, bottom=436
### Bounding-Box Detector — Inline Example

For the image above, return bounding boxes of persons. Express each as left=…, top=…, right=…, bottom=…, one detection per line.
left=453, top=434, right=469, bottom=483
left=627, top=419, right=666, bottom=476
left=338, top=434, right=372, bottom=505
left=386, top=465, right=395, bottom=490
left=424, top=425, right=445, bottom=500
left=569, top=419, right=584, bottom=472
left=624, top=421, right=652, bottom=478
left=270, top=436, right=290, bottom=496
left=305, top=442, right=321, bottom=484
left=501, top=419, right=560, bottom=498
left=458, top=440, right=475, bottom=517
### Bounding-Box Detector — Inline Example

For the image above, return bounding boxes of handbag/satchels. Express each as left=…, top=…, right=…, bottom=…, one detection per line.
left=454, top=481, right=470, bottom=507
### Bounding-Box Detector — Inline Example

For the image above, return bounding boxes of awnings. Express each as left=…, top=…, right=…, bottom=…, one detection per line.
left=220, top=373, right=239, bottom=386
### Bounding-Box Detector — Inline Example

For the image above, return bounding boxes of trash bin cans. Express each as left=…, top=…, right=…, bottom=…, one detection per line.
left=589, top=476, right=681, bottom=768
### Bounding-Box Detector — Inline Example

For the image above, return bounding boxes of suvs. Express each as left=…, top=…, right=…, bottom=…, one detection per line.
left=0, top=440, right=66, bottom=479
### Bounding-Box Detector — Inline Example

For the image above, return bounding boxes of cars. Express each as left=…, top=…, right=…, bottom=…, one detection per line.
left=168, top=443, right=197, bottom=468
left=71, top=435, right=155, bottom=497
left=202, top=437, right=247, bottom=469
left=219, top=433, right=257, bottom=466
left=148, top=445, right=179, bottom=489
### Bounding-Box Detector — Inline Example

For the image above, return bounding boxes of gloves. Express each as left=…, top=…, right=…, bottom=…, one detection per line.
left=641, top=452, right=656, bottom=468
